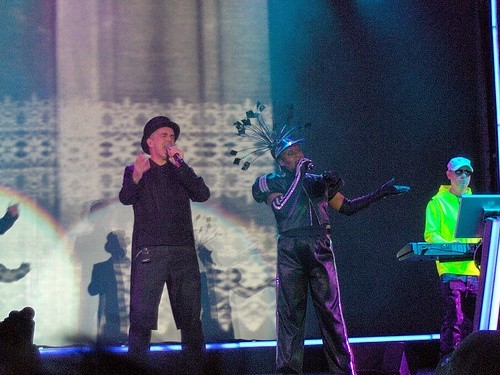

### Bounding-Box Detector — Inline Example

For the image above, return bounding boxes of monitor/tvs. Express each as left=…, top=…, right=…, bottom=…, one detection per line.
left=455, top=195, right=500, bottom=238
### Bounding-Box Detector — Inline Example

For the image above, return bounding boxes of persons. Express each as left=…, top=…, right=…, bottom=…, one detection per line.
left=120, top=116, right=207, bottom=363
left=251, top=136, right=410, bottom=375
left=424, top=157, right=484, bottom=359
left=0, top=202, right=21, bottom=234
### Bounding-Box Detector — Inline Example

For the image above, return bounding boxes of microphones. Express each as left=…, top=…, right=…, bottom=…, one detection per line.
left=305, top=162, right=314, bottom=170
left=164, top=144, right=183, bottom=163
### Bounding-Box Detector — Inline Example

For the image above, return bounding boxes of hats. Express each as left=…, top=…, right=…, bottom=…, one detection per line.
left=225, top=101, right=312, bottom=171
left=141, top=116, right=180, bottom=155
left=447, top=157, right=473, bottom=173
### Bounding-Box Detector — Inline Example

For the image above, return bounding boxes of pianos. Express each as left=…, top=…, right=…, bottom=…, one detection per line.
left=396, top=193, right=500, bottom=353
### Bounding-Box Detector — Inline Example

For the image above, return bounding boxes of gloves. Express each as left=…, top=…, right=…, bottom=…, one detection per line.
left=339, top=178, right=410, bottom=216
left=270, top=158, right=313, bottom=221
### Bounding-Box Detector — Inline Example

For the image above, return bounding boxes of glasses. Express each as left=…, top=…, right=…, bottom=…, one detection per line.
left=455, top=170, right=472, bottom=177
